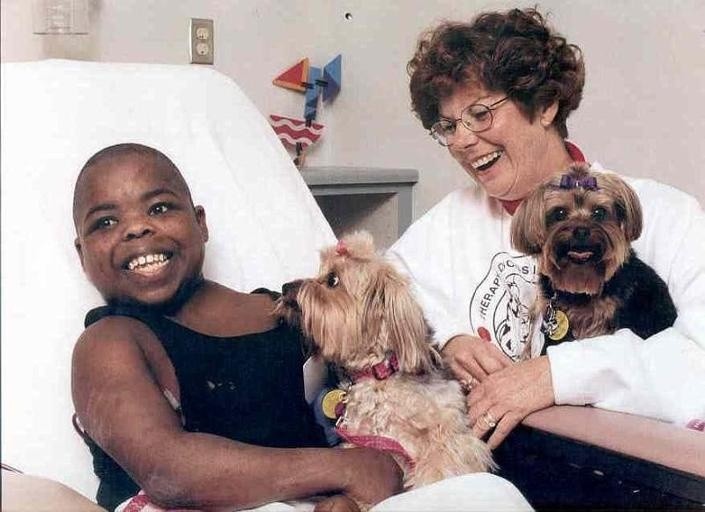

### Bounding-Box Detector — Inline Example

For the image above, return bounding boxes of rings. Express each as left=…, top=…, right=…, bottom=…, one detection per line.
left=482, top=411, right=496, bottom=427
left=459, top=373, right=474, bottom=393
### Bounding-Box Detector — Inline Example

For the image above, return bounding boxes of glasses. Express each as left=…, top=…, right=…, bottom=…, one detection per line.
left=428, top=95, right=512, bottom=148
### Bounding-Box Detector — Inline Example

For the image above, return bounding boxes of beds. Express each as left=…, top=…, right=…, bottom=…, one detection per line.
left=0, top=58, right=705, bottom=511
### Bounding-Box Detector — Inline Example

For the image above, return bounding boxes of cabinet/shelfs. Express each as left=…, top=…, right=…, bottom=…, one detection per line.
left=297, top=163, right=418, bottom=258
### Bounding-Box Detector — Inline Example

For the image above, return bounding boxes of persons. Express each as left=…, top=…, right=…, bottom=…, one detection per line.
left=383, top=6, right=705, bottom=452
left=69, top=142, right=538, bottom=512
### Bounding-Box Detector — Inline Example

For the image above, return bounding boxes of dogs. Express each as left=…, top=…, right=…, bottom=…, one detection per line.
left=509, top=161, right=678, bottom=362
left=265, top=228, right=501, bottom=512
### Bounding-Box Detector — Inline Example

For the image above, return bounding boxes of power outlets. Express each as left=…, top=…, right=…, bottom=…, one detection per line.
left=188, top=17, right=215, bottom=64
